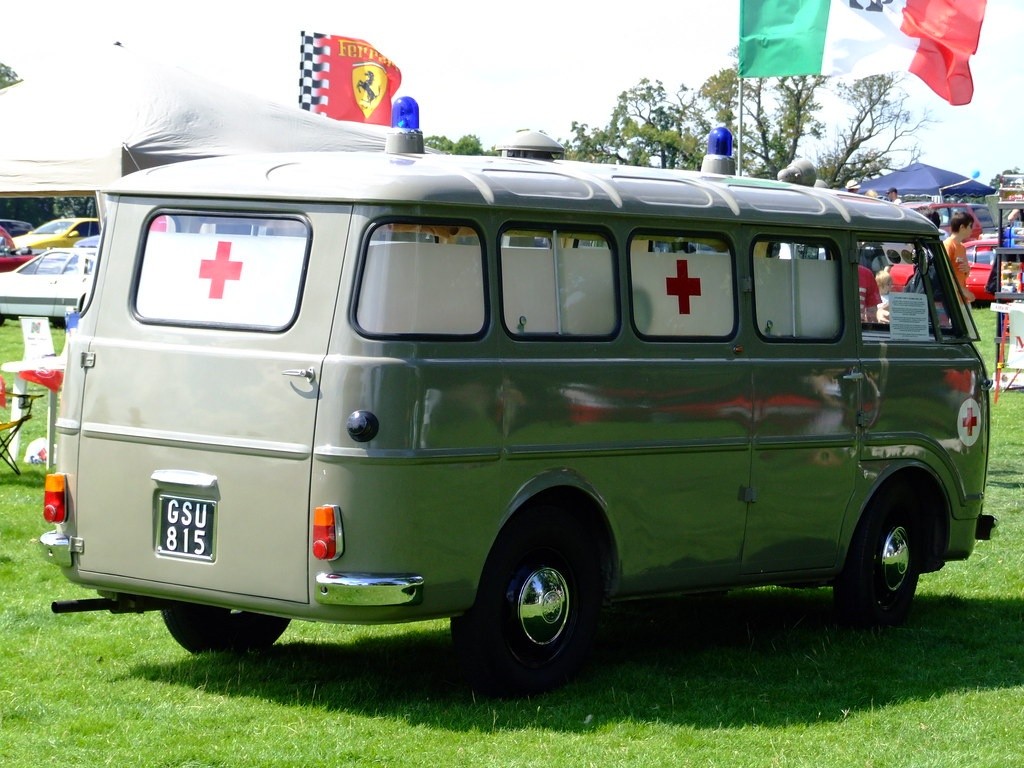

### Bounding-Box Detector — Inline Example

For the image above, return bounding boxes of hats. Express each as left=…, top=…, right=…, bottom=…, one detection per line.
left=885, top=187, right=898, bottom=194
left=845, top=179, right=861, bottom=190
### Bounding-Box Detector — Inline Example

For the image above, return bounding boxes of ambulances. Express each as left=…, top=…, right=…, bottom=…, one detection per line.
left=40, top=96, right=999, bottom=701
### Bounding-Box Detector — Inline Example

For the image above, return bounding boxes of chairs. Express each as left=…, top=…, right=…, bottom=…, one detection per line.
left=0, top=385, right=44, bottom=475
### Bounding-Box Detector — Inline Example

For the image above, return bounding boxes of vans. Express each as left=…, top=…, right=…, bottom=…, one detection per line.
left=898, top=202, right=1000, bottom=245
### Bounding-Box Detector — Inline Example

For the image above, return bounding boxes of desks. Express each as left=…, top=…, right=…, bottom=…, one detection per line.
left=0, top=357, right=67, bottom=470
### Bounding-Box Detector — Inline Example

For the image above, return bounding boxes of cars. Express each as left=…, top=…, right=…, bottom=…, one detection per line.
left=0, top=225, right=40, bottom=272
left=8, top=217, right=101, bottom=257
left=70, top=234, right=99, bottom=275
left=0, top=219, right=36, bottom=249
left=958, top=239, right=1024, bottom=309
left=0, top=247, right=98, bottom=328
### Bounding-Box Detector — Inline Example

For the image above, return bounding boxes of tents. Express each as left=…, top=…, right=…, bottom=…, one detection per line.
left=841, top=162, right=996, bottom=204
left=0, top=51, right=444, bottom=196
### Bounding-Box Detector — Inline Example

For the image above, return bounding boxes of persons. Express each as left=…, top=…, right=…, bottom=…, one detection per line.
left=857, top=187, right=976, bottom=324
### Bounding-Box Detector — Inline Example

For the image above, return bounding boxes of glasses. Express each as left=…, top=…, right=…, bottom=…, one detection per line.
left=967, top=224, right=973, bottom=229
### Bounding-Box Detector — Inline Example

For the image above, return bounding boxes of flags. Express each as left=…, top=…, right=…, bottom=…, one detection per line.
left=299, top=31, right=402, bottom=125
left=737, top=0, right=987, bottom=106
